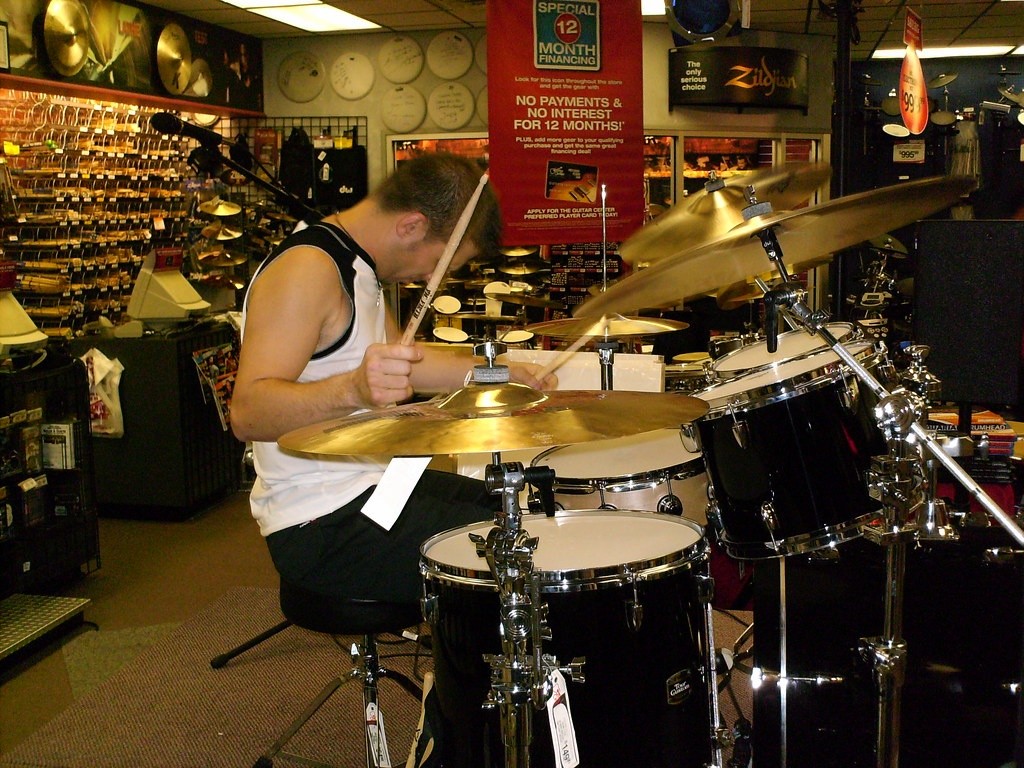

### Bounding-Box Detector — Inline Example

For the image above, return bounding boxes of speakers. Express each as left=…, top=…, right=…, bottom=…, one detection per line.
left=910, top=220, right=1024, bottom=425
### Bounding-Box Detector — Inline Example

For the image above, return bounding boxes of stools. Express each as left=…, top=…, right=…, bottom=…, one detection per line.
left=253, top=580, right=427, bottom=768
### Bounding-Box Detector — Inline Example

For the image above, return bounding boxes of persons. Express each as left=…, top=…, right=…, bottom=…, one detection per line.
left=232, top=148, right=559, bottom=608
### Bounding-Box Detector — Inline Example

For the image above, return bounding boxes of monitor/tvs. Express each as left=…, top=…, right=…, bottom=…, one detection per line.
left=0, top=290, right=48, bottom=360
left=128, top=267, right=211, bottom=320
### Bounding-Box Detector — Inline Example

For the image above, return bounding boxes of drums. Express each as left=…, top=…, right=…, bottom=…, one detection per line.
left=529, top=425, right=716, bottom=528
left=709, top=321, right=854, bottom=381
left=685, top=338, right=899, bottom=561
left=714, top=333, right=759, bottom=357
left=419, top=508, right=716, bottom=768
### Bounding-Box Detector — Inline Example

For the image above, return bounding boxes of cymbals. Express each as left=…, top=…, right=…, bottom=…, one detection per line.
left=277, top=380, right=712, bottom=458
left=929, top=110, right=957, bottom=125
left=499, top=246, right=538, bottom=257
left=873, top=246, right=910, bottom=259
left=987, top=70, right=1021, bottom=75
left=156, top=21, right=214, bottom=96
left=997, top=86, right=1021, bottom=103
left=43, top=0, right=90, bottom=78
left=197, top=250, right=248, bottom=267
left=927, top=72, right=959, bottom=89
left=615, top=161, right=834, bottom=268
left=200, top=200, right=242, bottom=216
left=881, top=93, right=901, bottom=115
left=200, top=224, right=243, bottom=241
left=571, top=172, right=982, bottom=318
left=498, top=264, right=540, bottom=275
left=851, top=75, right=883, bottom=85
left=522, top=312, right=690, bottom=338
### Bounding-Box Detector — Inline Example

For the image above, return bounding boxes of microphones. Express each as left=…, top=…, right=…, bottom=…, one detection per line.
left=150, top=113, right=242, bottom=149
